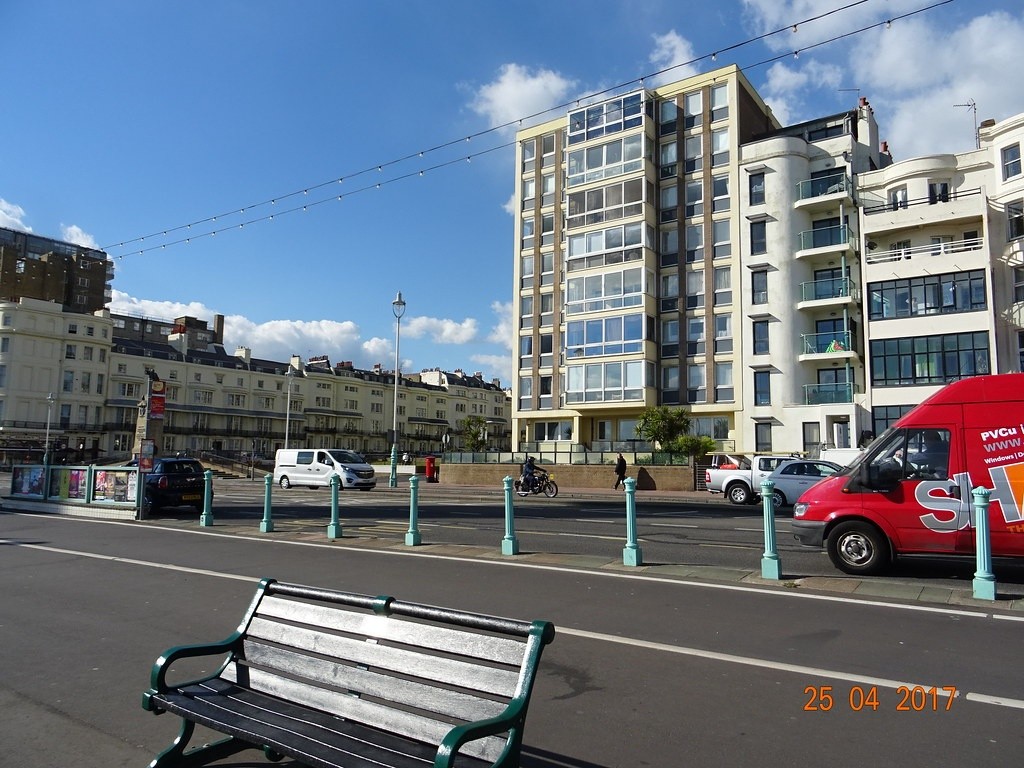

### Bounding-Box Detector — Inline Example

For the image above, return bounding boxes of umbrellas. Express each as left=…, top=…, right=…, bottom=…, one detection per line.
left=56, top=446, right=107, bottom=459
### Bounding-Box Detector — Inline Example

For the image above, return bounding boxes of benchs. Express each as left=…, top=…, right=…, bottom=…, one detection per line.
left=141, top=576, right=556, bottom=768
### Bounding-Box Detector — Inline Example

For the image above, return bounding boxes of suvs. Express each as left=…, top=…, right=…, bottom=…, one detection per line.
left=113, top=456, right=214, bottom=516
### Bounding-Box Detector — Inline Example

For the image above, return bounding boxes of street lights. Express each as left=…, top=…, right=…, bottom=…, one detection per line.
left=283, top=365, right=297, bottom=449
left=43, top=392, right=57, bottom=464
left=387, top=291, right=407, bottom=487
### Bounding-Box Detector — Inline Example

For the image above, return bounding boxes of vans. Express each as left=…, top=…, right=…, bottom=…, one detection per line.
left=272, top=446, right=378, bottom=492
left=790, top=372, right=1024, bottom=578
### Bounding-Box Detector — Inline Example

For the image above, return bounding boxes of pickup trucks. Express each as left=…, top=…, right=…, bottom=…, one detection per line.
left=704, top=452, right=820, bottom=505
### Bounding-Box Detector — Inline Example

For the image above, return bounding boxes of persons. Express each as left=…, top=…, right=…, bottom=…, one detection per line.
left=523, top=457, right=547, bottom=494
left=895, top=431, right=947, bottom=478
left=906, top=296, right=918, bottom=315
left=402, top=451, right=412, bottom=465
left=613, top=453, right=626, bottom=491
left=30, top=473, right=44, bottom=495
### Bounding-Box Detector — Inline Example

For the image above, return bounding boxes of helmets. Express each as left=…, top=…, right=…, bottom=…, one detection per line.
left=895, top=450, right=903, bottom=458
left=528, top=457, right=536, bottom=462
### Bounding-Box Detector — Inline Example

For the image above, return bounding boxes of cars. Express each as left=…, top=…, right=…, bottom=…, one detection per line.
left=241, top=451, right=275, bottom=467
left=760, top=460, right=846, bottom=513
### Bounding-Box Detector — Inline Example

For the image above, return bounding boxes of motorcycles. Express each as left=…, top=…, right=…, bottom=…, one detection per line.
left=514, top=469, right=559, bottom=498
